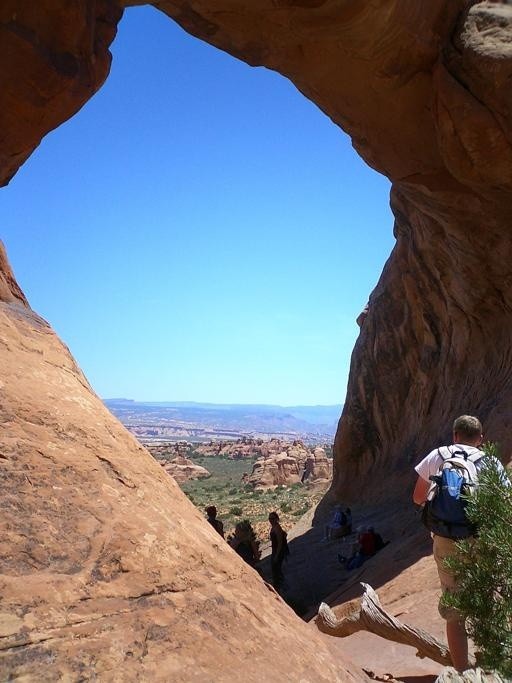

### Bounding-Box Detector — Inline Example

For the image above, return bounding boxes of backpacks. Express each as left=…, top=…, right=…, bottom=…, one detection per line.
left=422, top=446, right=486, bottom=539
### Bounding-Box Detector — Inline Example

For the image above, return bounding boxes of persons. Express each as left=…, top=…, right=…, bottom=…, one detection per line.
left=206, top=505, right=225, bottom=540
left=413, top=414, right=512, bottom=673
left=266, top=513, right=291, bottom=593
left=321, top=505, right=384, bottom=571
